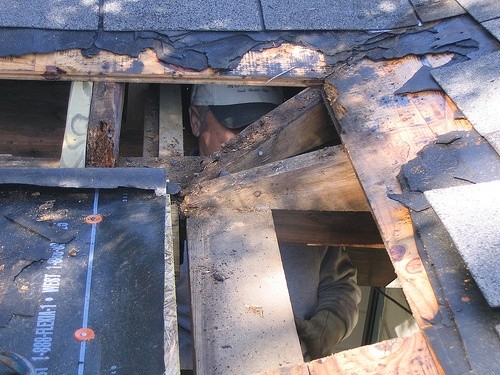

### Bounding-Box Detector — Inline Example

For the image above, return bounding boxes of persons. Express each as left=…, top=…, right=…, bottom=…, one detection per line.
left=175, top=84, right=365, bottom=372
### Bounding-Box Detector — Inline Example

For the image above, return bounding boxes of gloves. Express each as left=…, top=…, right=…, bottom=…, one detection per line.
left=293, top=309, right=348, bottom=362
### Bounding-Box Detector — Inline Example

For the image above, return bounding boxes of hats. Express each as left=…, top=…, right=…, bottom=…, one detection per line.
left=190, top=84, right=285, bottom=129
